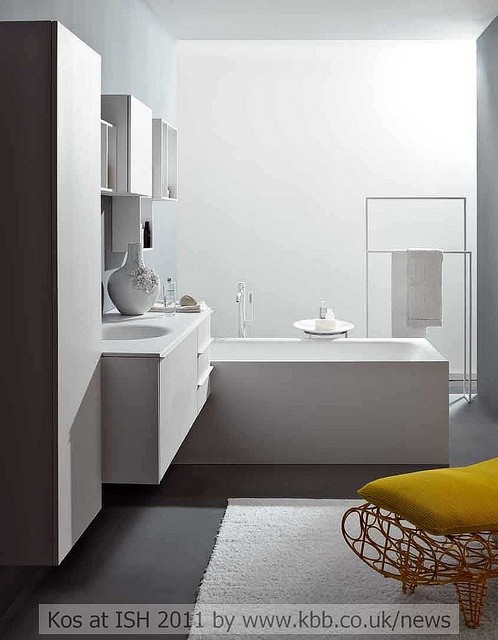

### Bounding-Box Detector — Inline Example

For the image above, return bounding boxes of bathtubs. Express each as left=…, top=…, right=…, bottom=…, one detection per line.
left=170, top=337, right=449, bottom=465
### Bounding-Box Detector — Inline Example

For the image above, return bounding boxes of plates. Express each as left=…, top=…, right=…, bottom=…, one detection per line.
left=292, top=319, right=355, bottom=334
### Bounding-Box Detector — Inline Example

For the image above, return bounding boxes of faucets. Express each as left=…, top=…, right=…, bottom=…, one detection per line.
left=236, top=282, right=244, bottom=338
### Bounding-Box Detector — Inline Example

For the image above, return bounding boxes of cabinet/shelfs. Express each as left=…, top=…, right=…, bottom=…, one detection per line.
left=197, top=308, right=215, bottom=415
left=102, top=328, right=198, bottom=486
left=100, top=118, right=118, bottom=197
left=100, top=92, right=154, bottom=199
left=111, top=196, right=155, bottom=253
left=0, top=17, right=105, bottom=570
left=151, top=117, right=178, bottom=204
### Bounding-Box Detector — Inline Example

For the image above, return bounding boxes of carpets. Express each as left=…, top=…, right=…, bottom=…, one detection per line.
left=189, top=497, right=498, bottom=639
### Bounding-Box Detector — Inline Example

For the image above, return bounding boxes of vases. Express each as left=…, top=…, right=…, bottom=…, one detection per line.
left=106, top=241, right=160, bottom=317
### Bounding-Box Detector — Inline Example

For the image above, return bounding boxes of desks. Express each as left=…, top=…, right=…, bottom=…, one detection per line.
left=292, top=317, right=356, bottom=339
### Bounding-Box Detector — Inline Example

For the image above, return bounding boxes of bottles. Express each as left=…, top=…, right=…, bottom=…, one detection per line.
left=163, top=278, right=176, bottom=318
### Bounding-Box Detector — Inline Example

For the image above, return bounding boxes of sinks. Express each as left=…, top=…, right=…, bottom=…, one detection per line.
left=101, top=323, right=171, bottom=340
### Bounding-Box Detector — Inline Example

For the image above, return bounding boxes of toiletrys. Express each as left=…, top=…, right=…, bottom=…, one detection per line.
left=142, top=225, right=145, bottom=248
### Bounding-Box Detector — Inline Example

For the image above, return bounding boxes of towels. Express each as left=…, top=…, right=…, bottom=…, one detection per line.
left=149, top=299, right=207, bottom=315
left=390, top=250, right=426, bottom=340
left=406, top=248, right=443, bottom=329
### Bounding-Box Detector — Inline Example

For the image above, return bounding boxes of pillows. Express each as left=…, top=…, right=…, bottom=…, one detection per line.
left=356, top=457, right=498, bottom=536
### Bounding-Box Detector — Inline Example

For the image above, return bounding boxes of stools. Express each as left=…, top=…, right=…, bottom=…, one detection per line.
left=341, top=457, right=498, bottom=630
